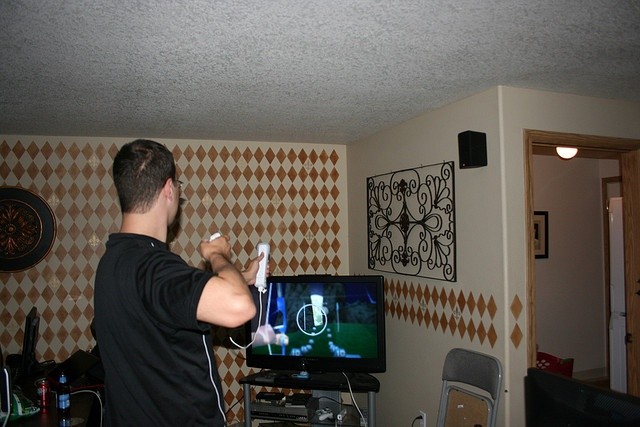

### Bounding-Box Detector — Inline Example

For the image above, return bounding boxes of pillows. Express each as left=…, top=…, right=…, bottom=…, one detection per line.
left=536, top=351, right=574, bottom=378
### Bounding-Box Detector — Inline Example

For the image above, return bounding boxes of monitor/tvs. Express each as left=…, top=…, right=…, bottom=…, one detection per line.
left=246, top=274, right=386, bottom=384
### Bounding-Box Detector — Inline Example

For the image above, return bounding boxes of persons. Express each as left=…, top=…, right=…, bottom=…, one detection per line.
left=93, top=139, right=256, bottom=427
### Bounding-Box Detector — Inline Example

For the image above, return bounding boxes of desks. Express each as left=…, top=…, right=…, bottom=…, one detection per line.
left=5, top=389, right=95, bottom=427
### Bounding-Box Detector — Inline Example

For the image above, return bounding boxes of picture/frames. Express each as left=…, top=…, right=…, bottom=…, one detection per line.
left=532, top=210, right=549, bottom=259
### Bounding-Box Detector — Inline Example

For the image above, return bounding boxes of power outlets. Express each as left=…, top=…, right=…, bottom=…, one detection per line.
left=419, top=410, right=427, bottom=427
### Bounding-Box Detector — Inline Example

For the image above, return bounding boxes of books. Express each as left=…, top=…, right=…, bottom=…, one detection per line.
left=285, top=393, right=312, bottom=408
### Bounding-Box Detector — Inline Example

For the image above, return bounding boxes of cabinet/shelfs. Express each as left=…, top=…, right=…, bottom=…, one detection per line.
left=237, top=370, right=381, bottom=427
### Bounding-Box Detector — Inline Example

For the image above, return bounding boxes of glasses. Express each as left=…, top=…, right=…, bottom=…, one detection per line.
left=162, top=177, right=183, bottom=188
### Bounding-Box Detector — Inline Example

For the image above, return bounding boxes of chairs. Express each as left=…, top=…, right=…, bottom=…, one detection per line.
left=435, top=347, right=504, bottom=427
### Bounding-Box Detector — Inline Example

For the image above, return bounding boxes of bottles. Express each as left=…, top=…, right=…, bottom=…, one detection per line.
left=56, top=365, right=72, bottom=415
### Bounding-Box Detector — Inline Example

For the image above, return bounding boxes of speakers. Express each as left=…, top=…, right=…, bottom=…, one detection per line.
left=458, top=129, right=487, bottom=169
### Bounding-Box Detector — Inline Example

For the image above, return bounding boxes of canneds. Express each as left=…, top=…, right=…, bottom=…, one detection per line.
left=36, top=379, right=50, bottom=413
